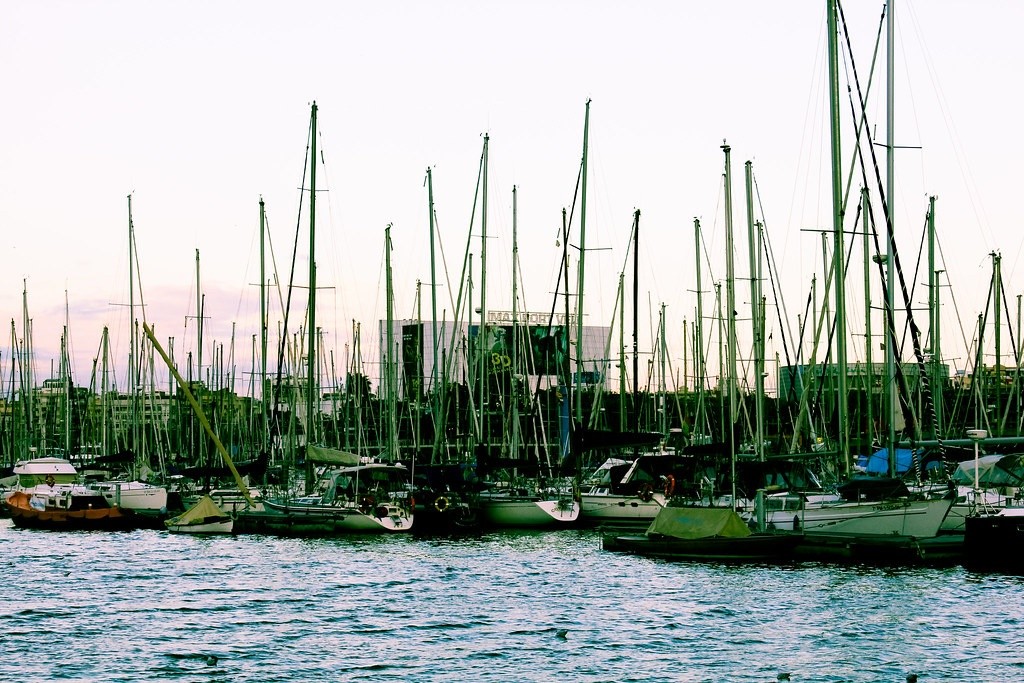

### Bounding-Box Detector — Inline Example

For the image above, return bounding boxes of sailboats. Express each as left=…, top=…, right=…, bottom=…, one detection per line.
left=0, top=0, right=1023, bottom=566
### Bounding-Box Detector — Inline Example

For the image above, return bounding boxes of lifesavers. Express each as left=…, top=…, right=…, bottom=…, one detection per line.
left=638, top=483, right=656, bottom=501
left=45, top=475, right=56, bottom=487
left=663, top=474, right=676, bottom=498
left=435, top=496, right=450, bottom=512
left=230, top=511, right=240, bottom=521
left=917, top=561, right=929, bottom=572
